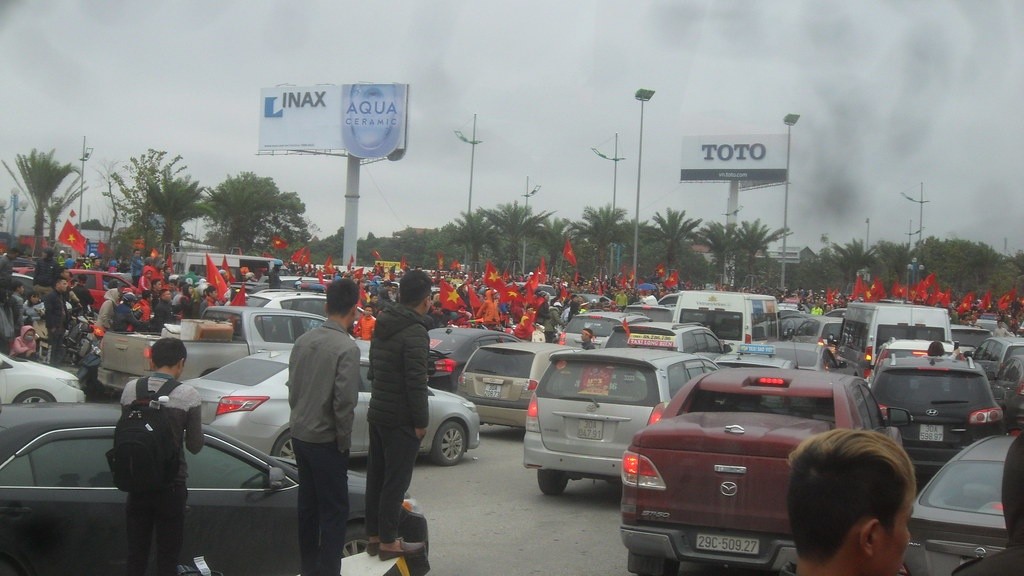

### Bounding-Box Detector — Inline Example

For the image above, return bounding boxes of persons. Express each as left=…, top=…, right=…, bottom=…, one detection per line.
left=785, top=430, right=917, bottom=576
left=927, top=341, right=960, bottom=392
left=678, top=280, right=740, bottom=292
left=580, top=328, right=595, bottom=350
left=287, top=278, right=362, bottom=576
left=365, top=270, right=434, bottom=560
left=0, top=248, right=281, bottom=363
left=740, top=286, right=853, bottom=315
left=119, top=338, right=205, bottom=576
left=285, top=262, right=677, bottom=338
left=950, top=299, right=1024, bottom=337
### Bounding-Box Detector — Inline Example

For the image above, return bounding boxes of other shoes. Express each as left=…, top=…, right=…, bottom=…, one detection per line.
left=367, top=540, right=381, bottom=556
left=379, top=537, right=425, bottom=560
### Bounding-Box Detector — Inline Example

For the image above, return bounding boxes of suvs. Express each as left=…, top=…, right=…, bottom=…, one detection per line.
left=247, top=288, right=377, bottom=337
left=521, top=347, right=724, bottom=496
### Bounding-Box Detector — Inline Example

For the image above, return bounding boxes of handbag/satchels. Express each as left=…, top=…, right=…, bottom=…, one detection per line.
left=174, top=295, right=190, bottom=319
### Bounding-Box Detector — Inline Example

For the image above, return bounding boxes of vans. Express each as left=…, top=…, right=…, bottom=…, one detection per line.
left=170, top=250, right=294, bottom=284
left=15, top=264, right=138, bottom=312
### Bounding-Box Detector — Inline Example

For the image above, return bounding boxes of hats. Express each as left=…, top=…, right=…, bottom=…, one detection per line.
left=245, top=273, right=254, bottom=280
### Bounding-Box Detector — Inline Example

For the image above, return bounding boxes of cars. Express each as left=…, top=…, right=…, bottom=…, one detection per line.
left=897, top=433, right=1024, bottom=576
left=7, top=273, right=74, bottom=317
left=229, top=274, right=328, bottom=294
left=455, top=340, right=588, bottom=430
left=0, top=400, right=431, bottom=576
left=428, top=327, right=524, bottom=392
left=504, top=280, right=1024, bottom=475
left=180, top=350, right=481, bottom=468
left=1, top=348, right=86, bottom=406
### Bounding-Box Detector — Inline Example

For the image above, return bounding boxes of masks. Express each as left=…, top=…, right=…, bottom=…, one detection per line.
left=23, top=335, right=35, bottom=343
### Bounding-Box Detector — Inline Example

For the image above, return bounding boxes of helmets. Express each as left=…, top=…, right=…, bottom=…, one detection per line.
left=122, top=291, right=137, bottom=301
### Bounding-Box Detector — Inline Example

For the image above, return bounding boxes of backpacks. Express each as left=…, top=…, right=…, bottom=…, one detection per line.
left=105, top=376, right=182, bottom=494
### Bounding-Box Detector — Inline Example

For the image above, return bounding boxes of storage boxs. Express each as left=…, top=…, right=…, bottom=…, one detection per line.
left=161, top=323, right=180, bottom=340
left=180, top=319, right=218, bottom=340
left=196, top=324, right=234, bottom=340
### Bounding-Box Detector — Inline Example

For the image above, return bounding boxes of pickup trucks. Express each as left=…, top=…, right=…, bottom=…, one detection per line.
left=95, top=304, right=355, bottom=393
left=618, top=363, right=913, bottom=576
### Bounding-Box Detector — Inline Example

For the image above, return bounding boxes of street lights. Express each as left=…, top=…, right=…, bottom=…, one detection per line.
left=906, top=257, right=925, bottom=285
left=0, top=187, right=29, bottom=249
left=904, top=219, right=926, bottom=254
left=589, top=130, right=628, bottom=280
left=632, top=88, right=656, bottom=288
left=865, top=218, right=870, bottom=251
left=780, top=112, right=802, bottom=289
left=720, top=197, right=744, bottom=284
left=899, top=180, right=933, bottom=283
left=520, top=175, right=541, bottom=274
left=452, top=113, right=484, bottom=274
left=74, top=134, right=95, bottom=259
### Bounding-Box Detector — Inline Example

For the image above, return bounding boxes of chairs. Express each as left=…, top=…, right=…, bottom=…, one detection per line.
left=258, top=319, right=273, bottom=341
left=720, top=318, right=739, bottom=332
left=950, top=376, right=971, bottom=400
left=889, top=374, right=911, bottom=400
left=962, top=483, right=996, bottom=510
left=919, top=377, right=945, bottom=401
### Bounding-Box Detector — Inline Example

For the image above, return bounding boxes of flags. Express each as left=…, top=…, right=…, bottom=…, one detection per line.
left=167, top=256, right=174, bottom=273
left=273, top=236, right=678, bottom=312
left=827, top=289, right=834, bottom=304
left=90, top=325, right=104, bottom=336
left=221, top=257, right=234, bottom=283
left=58, top=220, right=86, bottom=255
left=231, top=285, right=245, bottom=306
left=98, top=242, right=112, bottom=256
left=623, top=319, right=631, bottom=336
left=206, top=254, right=227, bottom=299
left=70, top=210, right=76, bottom=217
left=852, top=272, right=1018, bottom=318
left=150, top=249, right=158, bottom=259
